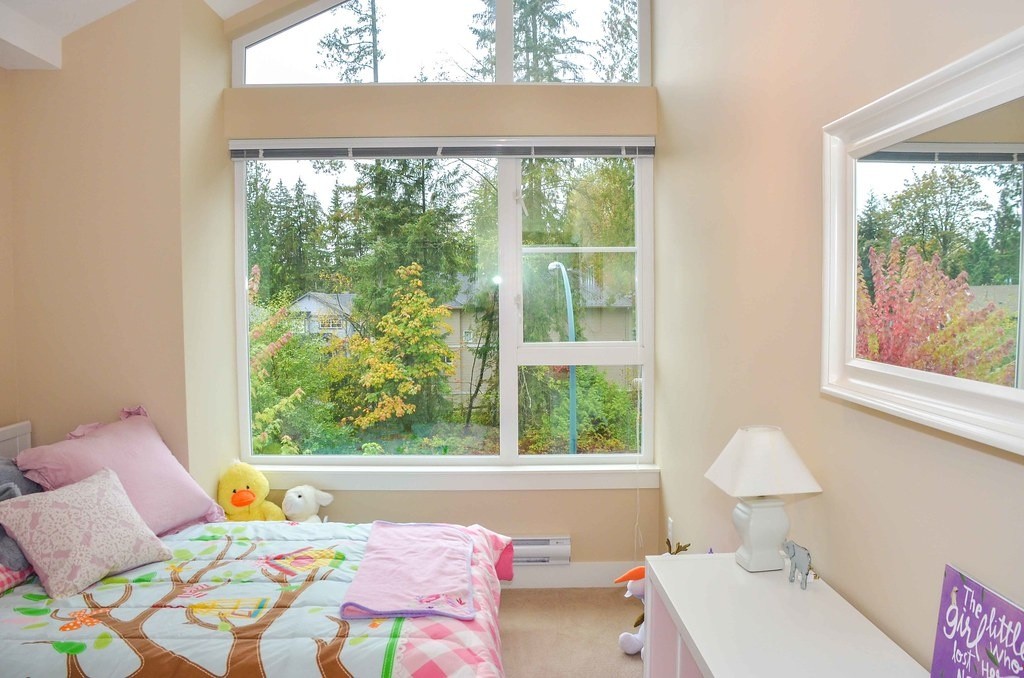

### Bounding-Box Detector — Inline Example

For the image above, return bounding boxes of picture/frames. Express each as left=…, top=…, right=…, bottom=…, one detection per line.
left=821, top=24, right=1024, bottom=463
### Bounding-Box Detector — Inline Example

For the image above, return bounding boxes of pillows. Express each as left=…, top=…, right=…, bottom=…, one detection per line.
left=0, top=468, right=173, bottom=597
left=14, top=404, right=227, bottom=541
left=1, top=457, right=44, bottom=596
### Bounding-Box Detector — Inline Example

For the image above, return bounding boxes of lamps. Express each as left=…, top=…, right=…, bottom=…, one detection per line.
left=703, top=424, right=823, bottom=573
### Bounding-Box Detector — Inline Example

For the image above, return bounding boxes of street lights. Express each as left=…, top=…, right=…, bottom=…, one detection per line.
left=547, top=261, right=577, bottom=455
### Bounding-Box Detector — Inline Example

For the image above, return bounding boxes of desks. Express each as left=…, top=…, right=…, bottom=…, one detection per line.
left=643, top=552, right=932, bottom=678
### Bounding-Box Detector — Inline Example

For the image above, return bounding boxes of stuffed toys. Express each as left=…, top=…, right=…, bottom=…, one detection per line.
left=218, top=464, right=286, bottom=523
left=282, top=485, right=334, bottom=525
left=614, top=564, right=646, bottom=661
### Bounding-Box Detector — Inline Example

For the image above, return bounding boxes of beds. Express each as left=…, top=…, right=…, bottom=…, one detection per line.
left=0, top=419, right=513, bottom=678
